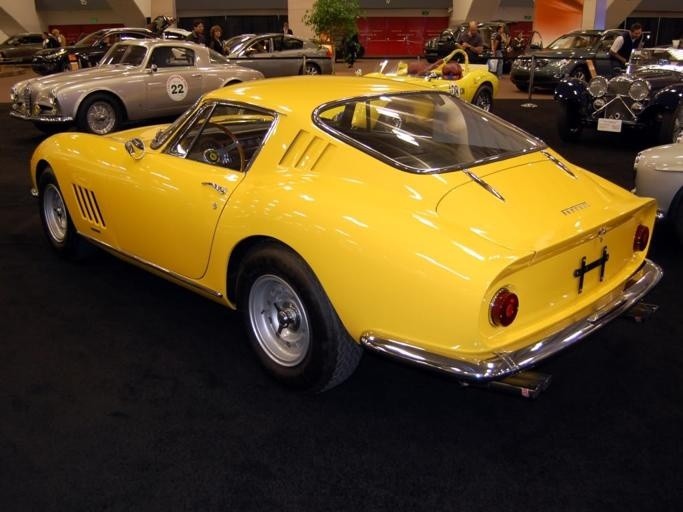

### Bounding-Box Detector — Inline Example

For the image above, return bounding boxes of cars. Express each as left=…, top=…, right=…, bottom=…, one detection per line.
left=627, top=139, right=683, bottom=248
left=26, top=74, right=666, bottom=399
left=10, top=40, right=265, bottom=136
left=360, top=49, right=499, bottom=115
left=224, top=32, right=335, bottom=77
left=31, top=16, right=176, bottom=76
left=0, top=33, right=47, bottom=64
left=509, top=28, right=652, bottom=94
left=423, top=21, right=512, bottom=64
left=552, top=44, right=683, bottom=151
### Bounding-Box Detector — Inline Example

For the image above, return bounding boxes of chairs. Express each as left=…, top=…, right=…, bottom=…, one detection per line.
left=442, top=62, right=462, bottom=80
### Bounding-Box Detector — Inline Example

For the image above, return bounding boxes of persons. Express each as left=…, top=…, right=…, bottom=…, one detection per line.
left=279, top=22, right=292, bottom=34
left=318, top=30, right=337, bottom=75
left=489, top=23, right=504, bottom=75
left=51, top=29, right=66, bottom=45
left=607, top=24, right=643, bottom=67
left=185, top=18, right=205, bottom=65
left=41, top=31, right=60, bottom=48
left=210, top=24, right=230, bottom=56
left=453, top=20, right=483, bottom=63
